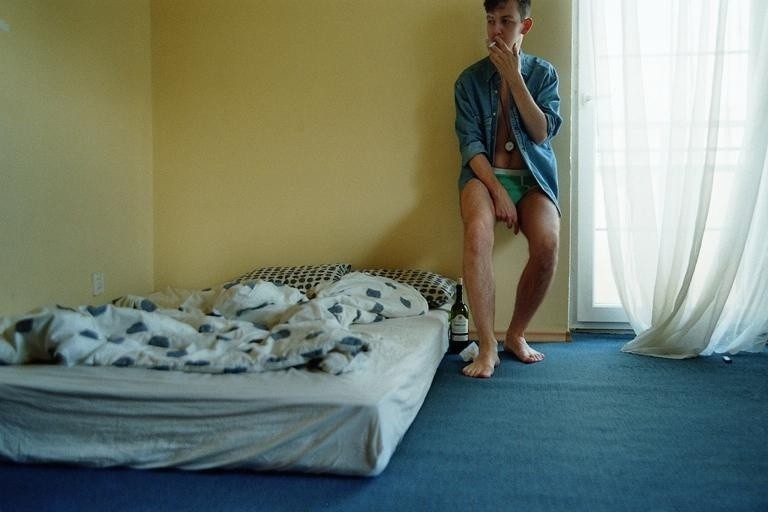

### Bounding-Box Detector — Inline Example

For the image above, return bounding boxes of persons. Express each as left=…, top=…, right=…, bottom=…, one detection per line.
left=453, top=0, right=564, bottom=380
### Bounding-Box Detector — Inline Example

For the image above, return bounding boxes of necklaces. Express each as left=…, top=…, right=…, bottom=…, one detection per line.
left=498, top=93, right=515, bottom=154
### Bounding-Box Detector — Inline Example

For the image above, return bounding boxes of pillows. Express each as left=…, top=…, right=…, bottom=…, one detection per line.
left=215, top=264, right=458, bottom=311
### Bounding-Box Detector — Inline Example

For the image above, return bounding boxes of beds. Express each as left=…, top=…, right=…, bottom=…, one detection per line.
left=2, top=289, right=464, bottom=478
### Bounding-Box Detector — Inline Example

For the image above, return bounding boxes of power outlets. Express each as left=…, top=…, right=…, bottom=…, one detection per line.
left=90, top=270, right=107, bottom=298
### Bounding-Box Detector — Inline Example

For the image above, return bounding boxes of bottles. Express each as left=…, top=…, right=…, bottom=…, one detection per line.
left=450, top=277, right=470, bottom=354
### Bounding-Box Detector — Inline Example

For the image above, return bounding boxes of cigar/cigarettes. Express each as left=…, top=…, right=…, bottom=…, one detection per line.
left=487, top=40, right=497, bottom=48
